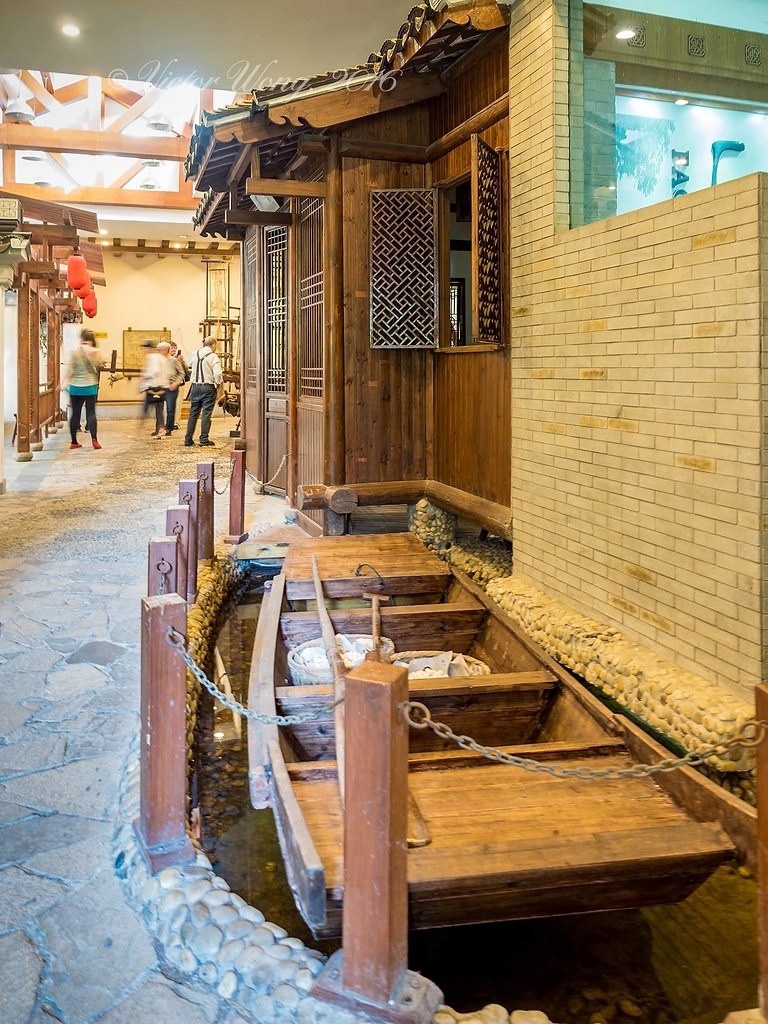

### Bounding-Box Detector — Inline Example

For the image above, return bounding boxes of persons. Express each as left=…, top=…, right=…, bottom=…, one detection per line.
left=126, top=339, right=192, bottom=440
left=54, top=329, right=104, bottom=448
left=184, top=336, right=223, bottom=446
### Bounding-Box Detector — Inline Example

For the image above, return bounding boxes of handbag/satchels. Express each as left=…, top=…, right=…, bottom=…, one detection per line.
left=184, top=371, right=191, bottom=382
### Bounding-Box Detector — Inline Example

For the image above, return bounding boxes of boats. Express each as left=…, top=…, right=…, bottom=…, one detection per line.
left=247, top=532, right=738, bottom=943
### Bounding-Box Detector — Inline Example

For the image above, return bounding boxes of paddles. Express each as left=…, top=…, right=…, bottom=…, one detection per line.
left=362, top=593, right=392, bottom=666
left=309, top=554, right=433, bottom=850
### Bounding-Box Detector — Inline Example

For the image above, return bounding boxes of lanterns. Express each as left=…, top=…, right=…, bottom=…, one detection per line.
left=67, top=251, right=97, bottom=318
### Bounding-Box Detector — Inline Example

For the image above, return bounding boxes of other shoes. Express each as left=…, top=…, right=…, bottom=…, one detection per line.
left=83, top=423, right=90, bottom=433
left=172, top=425, right=179, bottom=430
left=69, top=443, right=82, bottom=449
left=151, top=427, right=171, bottom=436
left=76, top=427, right=82, bottom=432
left=184, top=437, right=194, bottom=446
left=92, top=438, right=102, bottom=449
left=198, top=436, right=215, bottom=446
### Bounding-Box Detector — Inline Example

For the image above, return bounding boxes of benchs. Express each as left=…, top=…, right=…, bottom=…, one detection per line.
left=281, top=600, right=487, bottom=658
left=276, top=671, right=558, bottom=762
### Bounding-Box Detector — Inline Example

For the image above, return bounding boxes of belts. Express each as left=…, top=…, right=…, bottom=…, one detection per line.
left=192, top=383, right=213, bottom=385
